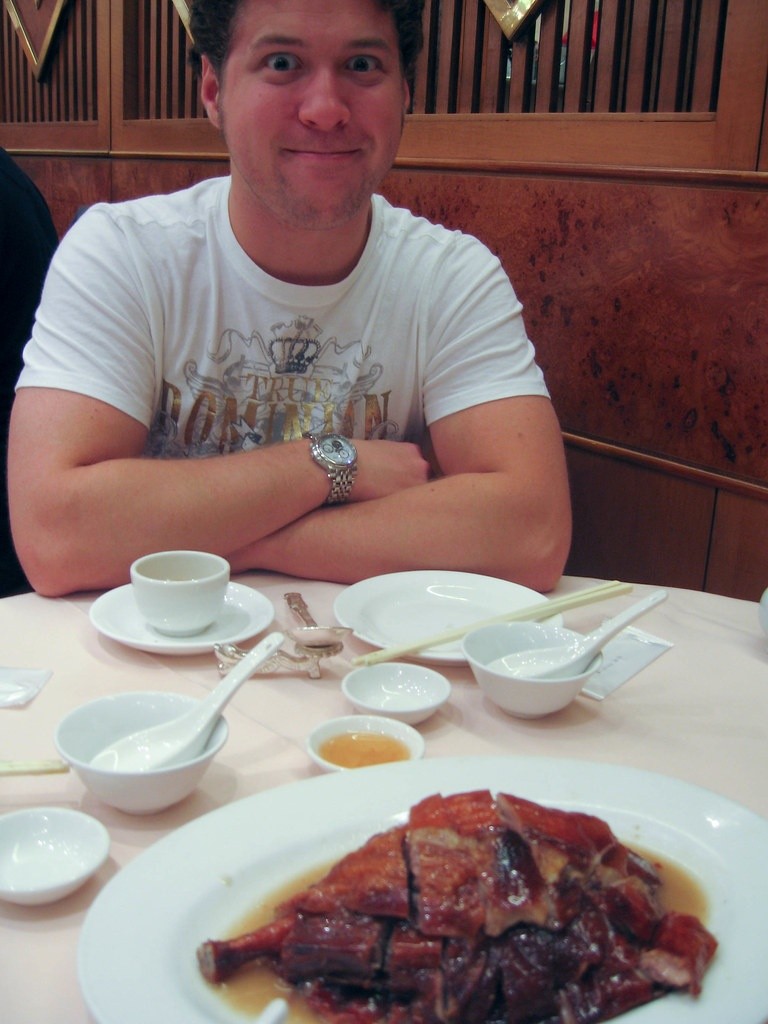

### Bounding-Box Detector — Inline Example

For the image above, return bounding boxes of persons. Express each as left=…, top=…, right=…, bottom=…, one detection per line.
left=8, top=1, right=571, bottom=599
left=0, top=144, right=61, bottom=600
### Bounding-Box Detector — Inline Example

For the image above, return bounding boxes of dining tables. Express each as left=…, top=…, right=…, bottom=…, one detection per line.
left=0, top=569, right=768, bottom=1024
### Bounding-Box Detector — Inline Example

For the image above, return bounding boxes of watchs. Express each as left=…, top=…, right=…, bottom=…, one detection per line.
left=304, top=432, right=357, bottom=506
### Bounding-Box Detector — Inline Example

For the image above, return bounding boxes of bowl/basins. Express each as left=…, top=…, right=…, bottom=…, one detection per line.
left=463, top=622, right=604, bottom=719
left=129, top=550, right=231, bottom=638
left=54, top=692, right=230, bottom=816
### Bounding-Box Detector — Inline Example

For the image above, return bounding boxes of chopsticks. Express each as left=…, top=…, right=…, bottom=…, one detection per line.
left=353, top=581, right=632, bottom=667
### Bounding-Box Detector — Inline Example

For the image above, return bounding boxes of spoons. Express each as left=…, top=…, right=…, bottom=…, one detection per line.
left=286, top=592, right=354, bottom=645
left=487, top=588, right=669, bottom=679
left=91, top=632, right=285, bottom=770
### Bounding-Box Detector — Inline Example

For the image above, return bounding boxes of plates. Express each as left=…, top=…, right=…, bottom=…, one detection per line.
left=307, top=715, right=426, bottom=771
left=90, top=581, right=275, bottom=656
left=79, top=755, right=768, bottom=1024
left=0, top=809, right=110, bottom=906
left=342, top=662, right=451, bottom=725
left=334, top=570, right=564, bottom=665
